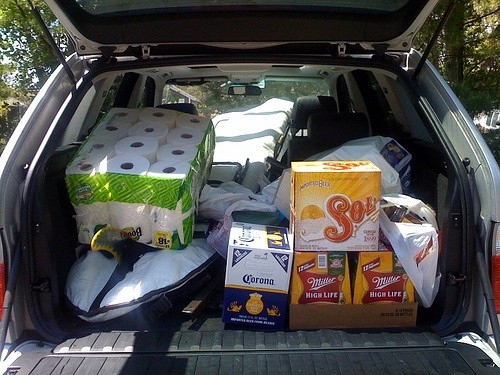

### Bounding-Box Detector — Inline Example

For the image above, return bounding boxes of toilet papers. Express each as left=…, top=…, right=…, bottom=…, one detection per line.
left=63, top=103, right=217, bottom=253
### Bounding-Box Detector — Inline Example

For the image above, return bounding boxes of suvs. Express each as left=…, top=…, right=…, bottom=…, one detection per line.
left=0, top=0, right=500, bottom=375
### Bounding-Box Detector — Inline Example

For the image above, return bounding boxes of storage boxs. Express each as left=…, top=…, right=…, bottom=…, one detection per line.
left=221, top=161, right=419, bottom=332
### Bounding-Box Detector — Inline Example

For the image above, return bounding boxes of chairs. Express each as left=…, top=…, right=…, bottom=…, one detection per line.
left=271, top=96, right=369, bottom=186
